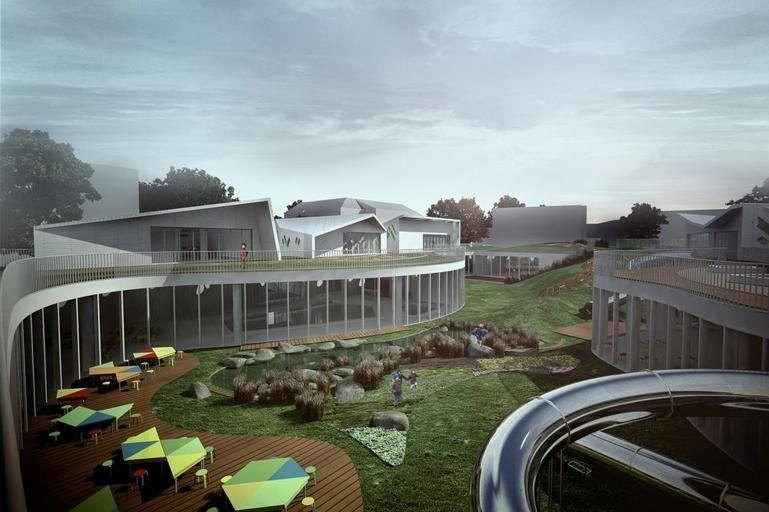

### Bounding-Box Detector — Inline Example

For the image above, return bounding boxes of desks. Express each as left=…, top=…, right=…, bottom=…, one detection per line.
left=133, top=345, right=175, bottom=366
left=223, top=457, right=308, bottom=512
left=60, top=406, right=136, bottom=441
left=56, top=387, right=92, bottom=408
left=90, top=360, right=142, bottom=390
left=121, top=427, right=206, bottom=493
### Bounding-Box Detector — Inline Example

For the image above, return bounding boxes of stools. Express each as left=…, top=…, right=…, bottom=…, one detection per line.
left=134, top=468, right=149, bottom=485
left=304, top=466, right=318, bottom=485
left=131, top=413, right=142, bottom=428
left=302, top=497, right=317, bottom=512
left=196, top=468, right=210, bottom=489
left=219, top=474, right=233, bottom=486
left=168, top=357, right=177, bottom=368
left=177, top=350, right=186, bottom=361
left=147, top=368, right=157, bottom=382
left=140, top=361, right=150, bottom=371
left=131, top=378, right=141, bottom=392
left=60, top=404, right=72, bottom=413
left=91, top=428, right=104, bottom=445
left=48, top=431, right=61, bottom=446
left=102, top=459, right=115, bottom=476
left=205, top=447, right=216, bottom=465
left=101, top=380, right=113, bottom=391
left=50, top=417, right=60, bottom=431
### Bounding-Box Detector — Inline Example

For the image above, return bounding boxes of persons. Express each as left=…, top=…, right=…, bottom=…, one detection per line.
left=343, top=240, right=350, bottom=253
left=239, top=242, right=249, bottom=272
left=350, top=239, right=359, bottom=251
left=389, top=370, right=407, bottom=405
left=407, top=368, right=422, bottom=390
left=474, top=323, right=488, bottom=347
